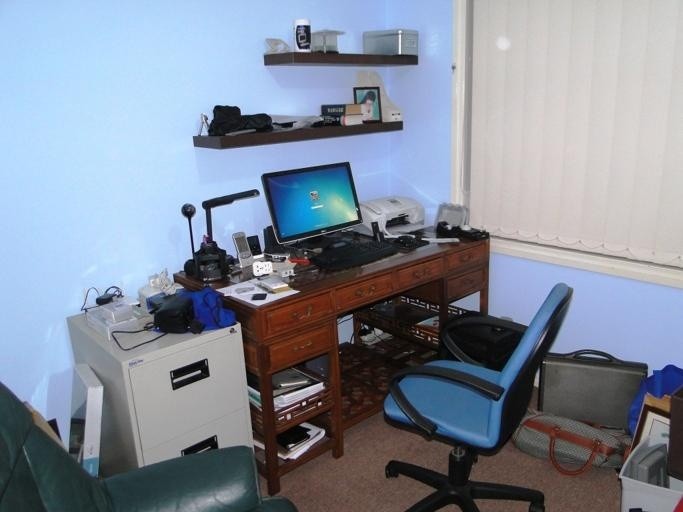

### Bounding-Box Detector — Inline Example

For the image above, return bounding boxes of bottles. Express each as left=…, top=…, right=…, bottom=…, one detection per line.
left=293, top=20, right=311, bottom=54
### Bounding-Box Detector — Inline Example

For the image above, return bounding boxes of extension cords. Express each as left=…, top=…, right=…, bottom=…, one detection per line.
left=360, top=328, right=383, bottom=342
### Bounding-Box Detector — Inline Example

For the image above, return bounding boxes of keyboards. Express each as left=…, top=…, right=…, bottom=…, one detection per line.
left=312, top=240, right=397, bottom=272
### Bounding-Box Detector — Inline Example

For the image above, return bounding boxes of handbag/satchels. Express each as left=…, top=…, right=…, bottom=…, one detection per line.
left=511, top=408, right=632, bottom=476
left=112, top=297, right=205, bottom=352
left=174, top=288, right=235, bottom=331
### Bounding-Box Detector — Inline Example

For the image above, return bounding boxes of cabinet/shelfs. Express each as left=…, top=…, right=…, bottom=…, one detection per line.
left=190, top=51, right=419, bottom=151
left=65, top=304, right=255, bottom=468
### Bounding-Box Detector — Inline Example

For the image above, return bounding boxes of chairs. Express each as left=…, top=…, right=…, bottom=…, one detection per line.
left=0, top=381, right=299, bottom=510
left=381, top=280, right=573, bottom=512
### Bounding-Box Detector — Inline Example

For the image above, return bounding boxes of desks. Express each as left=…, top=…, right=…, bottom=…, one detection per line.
left=171, top=223, right=490, bottom=498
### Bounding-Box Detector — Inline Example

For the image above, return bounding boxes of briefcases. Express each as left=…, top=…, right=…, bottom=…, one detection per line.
left=537, top=349, right=647, bottom=433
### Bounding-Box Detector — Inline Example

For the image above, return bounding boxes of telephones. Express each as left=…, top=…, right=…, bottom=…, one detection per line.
left=232, top=232, right=254, bottom=269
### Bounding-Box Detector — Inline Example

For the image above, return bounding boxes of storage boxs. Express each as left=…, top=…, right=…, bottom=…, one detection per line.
left=617, top=437, right=683, bottom=512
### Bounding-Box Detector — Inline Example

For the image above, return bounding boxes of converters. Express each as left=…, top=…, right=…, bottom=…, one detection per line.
left=358, top=329, right=370, bottom=336
left=95, top=293, right=116, bottom=305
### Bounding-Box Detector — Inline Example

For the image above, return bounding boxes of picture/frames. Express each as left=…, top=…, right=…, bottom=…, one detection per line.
left=353, top=86, right=381, bottom=124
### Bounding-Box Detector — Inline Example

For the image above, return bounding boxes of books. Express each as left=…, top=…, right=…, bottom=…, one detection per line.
left=67, top=363, right=104, bottom=479
left=319, top=114, right=363, bottom=127
left=320, top=103, right=361, bottom=115
left=630, top=442, right=670, bottom=487
left=246, top=365, right=327, bottom=461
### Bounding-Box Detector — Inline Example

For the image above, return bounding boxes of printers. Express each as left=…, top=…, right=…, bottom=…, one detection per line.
left=353, top=196, right=425, bottom=238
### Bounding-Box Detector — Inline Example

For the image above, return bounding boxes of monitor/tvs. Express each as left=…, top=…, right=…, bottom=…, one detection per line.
left=260, top=162, right=364, bottom=252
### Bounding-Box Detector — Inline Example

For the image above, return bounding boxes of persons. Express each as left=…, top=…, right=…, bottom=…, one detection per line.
left=358, top=90, right=381, bottom=120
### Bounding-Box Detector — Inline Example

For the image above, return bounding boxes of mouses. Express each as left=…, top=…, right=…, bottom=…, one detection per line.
left=397, top=236, right=417, bottom=247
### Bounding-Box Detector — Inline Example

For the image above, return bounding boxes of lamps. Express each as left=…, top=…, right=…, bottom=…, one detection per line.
left=180, top=189, right=260, bottom=274
